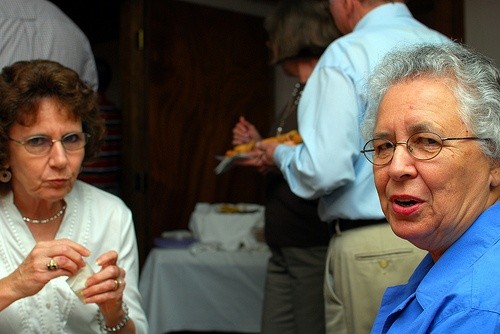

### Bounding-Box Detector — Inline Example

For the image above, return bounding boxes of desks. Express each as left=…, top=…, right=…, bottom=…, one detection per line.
left=139, top=233, right=270, bottom=334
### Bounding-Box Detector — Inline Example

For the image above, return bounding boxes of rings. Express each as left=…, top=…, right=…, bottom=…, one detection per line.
left=114, top=279, right=121, bottom=290
left=48, top=258, right=57, bottom=270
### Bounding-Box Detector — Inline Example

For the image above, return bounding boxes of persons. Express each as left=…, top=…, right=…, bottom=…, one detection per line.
left=0, top=59, right=150, bottom=334
left=77, top=85, right=125, bottom=200
left=231, top=0, right=337, bottom=334
left=253, top=0, right=470, bottom=334
left=358, top=38, right=499, bottom=333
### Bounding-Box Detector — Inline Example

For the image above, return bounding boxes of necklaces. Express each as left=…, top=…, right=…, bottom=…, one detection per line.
left=276, top=84, right=306, bottom=134
left=22, top=205, right=67, bottom=224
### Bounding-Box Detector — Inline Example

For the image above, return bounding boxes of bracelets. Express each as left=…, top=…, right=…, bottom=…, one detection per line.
left=97, top=301, right=130, bottom=333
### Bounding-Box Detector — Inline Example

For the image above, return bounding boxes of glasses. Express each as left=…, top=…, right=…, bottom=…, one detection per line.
left=360, top=132, right=492, bottom=166
left=5, top=131, right=89, bottom=155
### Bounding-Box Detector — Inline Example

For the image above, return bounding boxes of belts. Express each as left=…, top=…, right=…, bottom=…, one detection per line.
left=330, top=217, right=387, bottom=234
